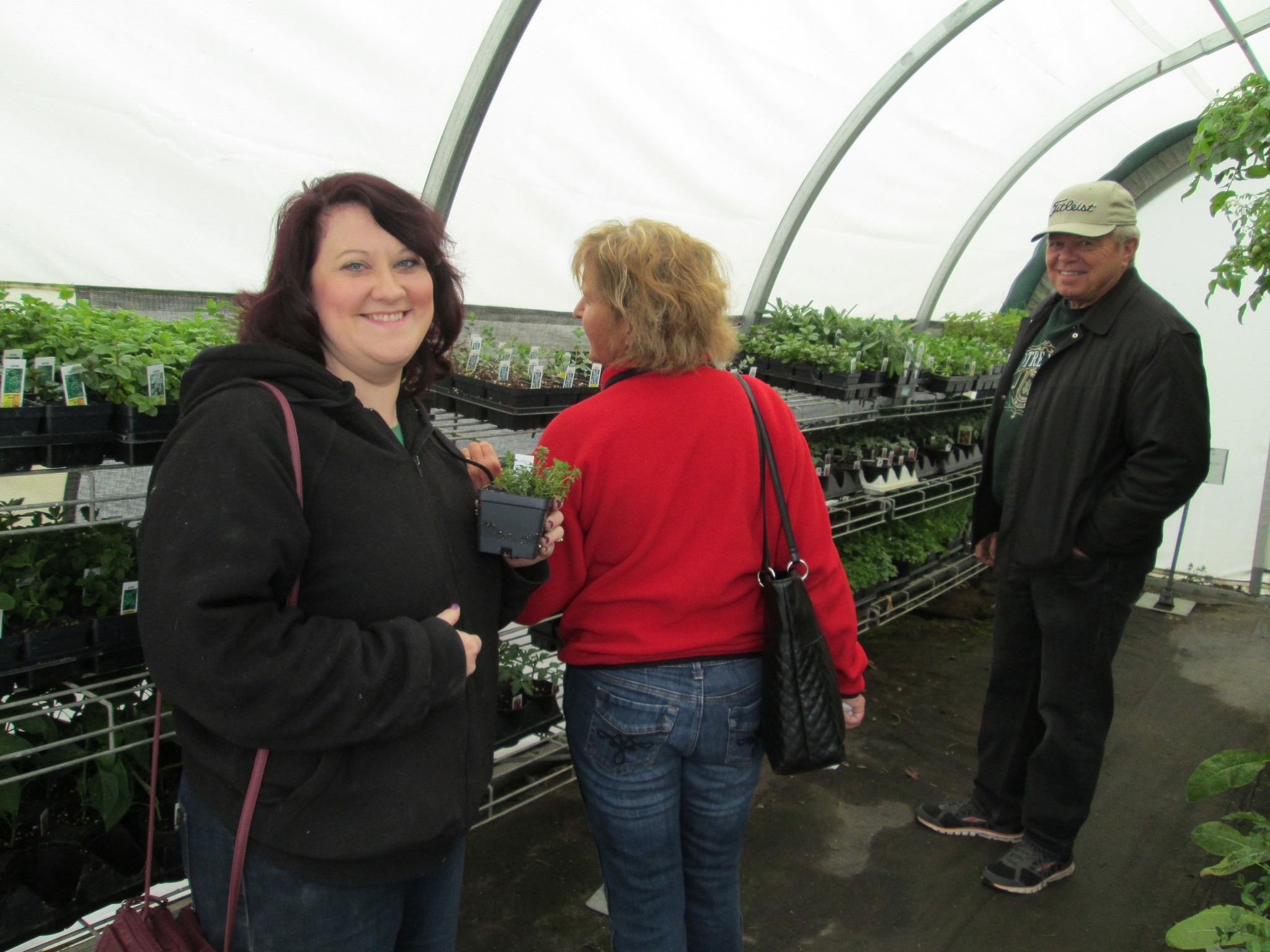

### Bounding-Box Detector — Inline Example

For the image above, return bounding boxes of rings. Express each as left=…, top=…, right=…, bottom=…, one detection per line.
left=555, top=538, right=563, bottom=542
left=466, top=457, right=471, bottom=460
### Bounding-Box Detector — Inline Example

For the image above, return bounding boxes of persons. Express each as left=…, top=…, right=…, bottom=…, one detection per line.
left=461, top=220, right=867, bottom=952
left=915, top=181, right=1210, bottom=892
left=135, top=176, right=564, bottom=952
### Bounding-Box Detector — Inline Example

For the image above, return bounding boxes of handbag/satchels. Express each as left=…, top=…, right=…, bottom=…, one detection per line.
left=764, top=571, right=845, bottom=775
left=95, top=907, right=215, bottom=952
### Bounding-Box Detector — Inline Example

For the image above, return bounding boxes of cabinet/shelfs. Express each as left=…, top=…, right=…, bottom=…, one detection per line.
left=1, top=393, right=1000, bottom=952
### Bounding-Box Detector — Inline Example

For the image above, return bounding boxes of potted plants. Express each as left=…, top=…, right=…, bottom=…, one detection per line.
left=0, top=306, right=1035, bottom=952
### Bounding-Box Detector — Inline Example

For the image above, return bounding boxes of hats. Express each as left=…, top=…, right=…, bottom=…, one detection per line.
left=1031, top=180, right=1137, bottom=243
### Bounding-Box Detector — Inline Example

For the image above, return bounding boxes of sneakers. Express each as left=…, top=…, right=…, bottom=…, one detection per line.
left=914, top=797, right=1024, bottom=840
left=979, top=840, right=1074, bottom=894
